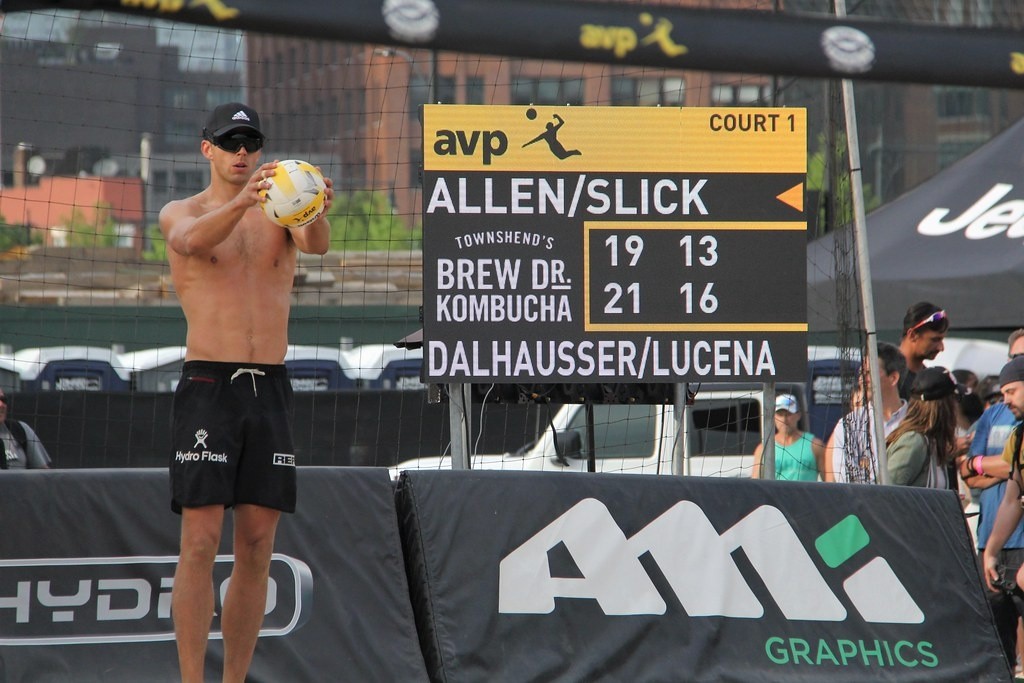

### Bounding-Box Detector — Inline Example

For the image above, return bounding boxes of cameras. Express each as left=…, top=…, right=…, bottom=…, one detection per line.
left=991, top=564, right=1020, bottom=592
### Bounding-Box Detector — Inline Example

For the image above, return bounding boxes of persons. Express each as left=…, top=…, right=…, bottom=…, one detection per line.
left=160, top=102, right=334, bottom=683
left=0, top=386, right=53, bottom=469
left=752, top=395, right=826, bottom=482
left=824, top=303, right=1024, bottom=677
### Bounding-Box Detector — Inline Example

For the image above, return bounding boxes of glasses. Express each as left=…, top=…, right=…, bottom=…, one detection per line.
left=207, top=133, right=263, bottom=153
left=911, top=310, right=947, bottom=330
left=1008, top=353, right=1024, bottom=359
left=989, top=397, right=1004, bottom=405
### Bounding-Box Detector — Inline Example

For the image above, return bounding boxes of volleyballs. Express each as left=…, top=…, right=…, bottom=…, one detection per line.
left=257, top=159, right=329, bottom=229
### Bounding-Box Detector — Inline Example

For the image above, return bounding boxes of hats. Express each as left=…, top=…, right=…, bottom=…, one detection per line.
left=911, top=366, right=967, bottom=400
left=205, top=103, right=266, bottom=138
left=775, top=394, right=799, bottom=414
left=985, top=384, right=1002, bottom=401
left=998, top=356, right=1024, bottom=388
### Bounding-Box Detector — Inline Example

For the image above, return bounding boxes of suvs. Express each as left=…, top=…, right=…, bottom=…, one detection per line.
left=388, top=339, right=860, bottom=483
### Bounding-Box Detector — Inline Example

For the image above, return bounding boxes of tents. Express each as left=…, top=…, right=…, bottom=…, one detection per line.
left=806, top=113, right=1024, bottom=332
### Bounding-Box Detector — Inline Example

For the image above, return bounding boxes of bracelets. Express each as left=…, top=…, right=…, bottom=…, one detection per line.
left=975, top=455, right=984, bottom=477
left=967, top=456, right=978, bottom=476
